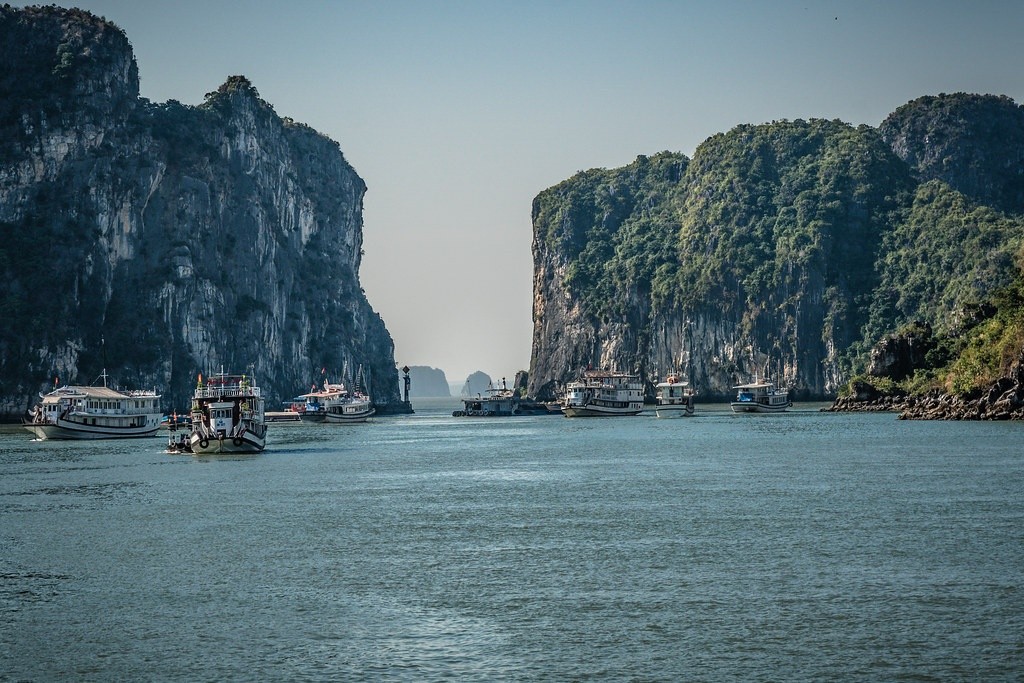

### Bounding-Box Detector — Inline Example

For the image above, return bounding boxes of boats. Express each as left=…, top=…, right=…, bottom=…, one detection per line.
left=190, top=362, right=268, bottom=453
left=283, top=361, right=376, bottom=423
left=653, top=353, right=695, bottom=419
left=452, top=376, right=524, bottom=417
left=544, top=360, right=644, bottom=417
left=168, top=416, right=192, bottom=424
left=731, top=356, right=793, bottom=413
left=169, top=432, right=191, bottom=453
left=21, top=331, right=164, bottom=439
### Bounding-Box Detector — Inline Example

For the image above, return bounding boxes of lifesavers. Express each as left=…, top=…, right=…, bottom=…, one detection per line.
left=452, top=410, right=497, bottom=417
left=199, top=440, right=209, bottom=448
left=233, top=437, right=243, bottom=447
left=789, top=403, right=793, bottom=407
